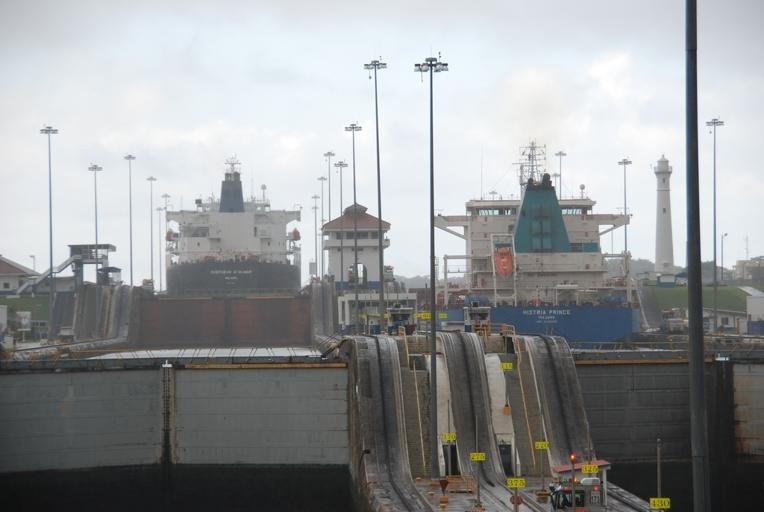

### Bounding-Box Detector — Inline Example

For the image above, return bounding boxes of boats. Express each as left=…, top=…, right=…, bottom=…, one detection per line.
left=496, top=250, right=512, bottom=277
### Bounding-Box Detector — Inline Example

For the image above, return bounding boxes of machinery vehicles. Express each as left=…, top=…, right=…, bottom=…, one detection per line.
left=546, top=477, right=606, bottom=511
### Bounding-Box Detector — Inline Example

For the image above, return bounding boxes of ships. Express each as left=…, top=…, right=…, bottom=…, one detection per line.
left=164, top=154, right=302, bottom=295
left=412, top=139, right=640, bottom=344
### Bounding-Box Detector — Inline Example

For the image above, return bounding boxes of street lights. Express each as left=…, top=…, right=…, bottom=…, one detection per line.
left=29, top=254, right=35, bottom=271
left=488, top=190, right=497, bottom=215
left=38, top=125, right=57, bottom=339
left=554, top=150, right=567, bottom=201
left=311, top=122, right=362, bottom=286
left=705, top=116, right=723, bottom=337
left=86, top=153, right=171, bottom=295
left=412, top=55, right=449, bottom=477
left=617, top=158, right=632, bottom=252
left=569, top=453, right=576, bottom=512
left=551, top=173, right=560, bottom=191
left=365, top=57, right=387, bottom=338
left=719, top=232, right=728, bottom=281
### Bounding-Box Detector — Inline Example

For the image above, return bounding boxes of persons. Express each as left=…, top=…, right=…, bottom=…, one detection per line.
left=560, top=494, right=584, bottom=506
